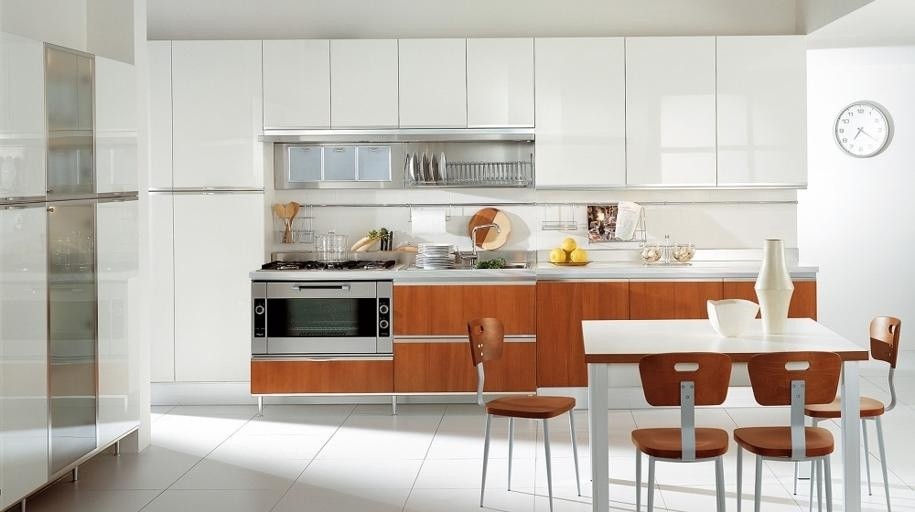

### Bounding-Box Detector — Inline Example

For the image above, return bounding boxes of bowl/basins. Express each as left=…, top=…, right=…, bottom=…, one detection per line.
left=707, top=296, right=759, bottom=339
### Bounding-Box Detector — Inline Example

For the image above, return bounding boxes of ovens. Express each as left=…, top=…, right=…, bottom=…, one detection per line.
left=250, top=280, right=393, bottom=357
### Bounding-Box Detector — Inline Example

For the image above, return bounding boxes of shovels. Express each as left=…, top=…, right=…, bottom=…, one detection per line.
left=274, top=202, right=300, bottom=242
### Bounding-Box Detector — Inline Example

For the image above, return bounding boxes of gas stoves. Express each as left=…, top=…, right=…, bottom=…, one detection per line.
left=261, top=260, right=396, bottom=272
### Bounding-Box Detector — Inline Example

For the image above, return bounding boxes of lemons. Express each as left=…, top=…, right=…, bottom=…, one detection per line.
left=549, top=248, right=566, bottom=262
left=570, top=249, right=589, bottom=262
left=562, top=238, right=577, bottom=252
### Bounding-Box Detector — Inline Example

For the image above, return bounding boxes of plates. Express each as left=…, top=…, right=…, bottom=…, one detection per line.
left=550, top=261, right=594, bottom=268
left=407, top=153, right=451, bottom=182
left=414, top=244, right=458, bottom=271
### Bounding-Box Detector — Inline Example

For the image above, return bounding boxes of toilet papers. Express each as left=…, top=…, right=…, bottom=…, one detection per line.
left=411, top=208, right=447, bottom=234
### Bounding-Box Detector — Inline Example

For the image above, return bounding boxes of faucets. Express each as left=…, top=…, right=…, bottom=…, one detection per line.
left=470, top=222, right=501, bottom=265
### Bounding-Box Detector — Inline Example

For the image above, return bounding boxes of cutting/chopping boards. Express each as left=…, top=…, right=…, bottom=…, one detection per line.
left=468, top=207, right=513, bottom=250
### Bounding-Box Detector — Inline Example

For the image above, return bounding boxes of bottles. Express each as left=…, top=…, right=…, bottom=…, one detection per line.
left=751, top=237, right=795, bottom=337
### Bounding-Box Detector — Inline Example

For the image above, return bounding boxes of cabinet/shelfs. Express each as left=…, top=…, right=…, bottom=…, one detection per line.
left=393, top=276, right=537, bottom=395
left=399, top=38, right=467, bottom=129
left=467, top=37, right=534, bottom=128
left=148, top=40, right=263, bottom=191
left=717, top=35, right=807, bottom=186
left=723, top=277, right=816, bottom=386
left=629, top=278, right=723, bottom=387
left=537, top=278, right=629, bottom=387
left=330, top=39, right=399, bottom=130
left=626, top=36, right=717, bottom=187
left=150, top=194, right=264, bottom=382
left=534, top=37, right=626, bottom=187
left=263, top=39, right=330, bottom=130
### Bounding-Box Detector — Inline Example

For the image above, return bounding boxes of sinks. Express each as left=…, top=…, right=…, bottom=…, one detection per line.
left=473, top=261, right=527, bottom=270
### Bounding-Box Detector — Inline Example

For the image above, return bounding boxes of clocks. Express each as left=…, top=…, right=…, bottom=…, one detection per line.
left=835, top=101, right=890, bottom=158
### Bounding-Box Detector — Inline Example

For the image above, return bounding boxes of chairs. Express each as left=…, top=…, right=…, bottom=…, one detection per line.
left=631, top=352, right=731, bottom=512
left=804, top=316, right=901, bottom=512
left=734, top=352, right=841, bottom=512
left=467, top=317, right=581, bottom=511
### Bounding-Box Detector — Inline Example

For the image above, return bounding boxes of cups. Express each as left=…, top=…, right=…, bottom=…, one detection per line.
left=673, top=240, right=695, bottom=262
left=641, top=242, right=663, bottom=262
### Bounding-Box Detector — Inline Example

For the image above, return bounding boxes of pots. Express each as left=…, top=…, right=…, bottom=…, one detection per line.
left=313, top=231, right=349, bottom=265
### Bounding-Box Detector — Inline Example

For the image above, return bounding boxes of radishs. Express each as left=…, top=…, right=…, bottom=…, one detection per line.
left=355, top=237, right=378, bottom=253
left=394, top=246, right=418, bottom=253
left=350, top=235, right=370, bottom=251
